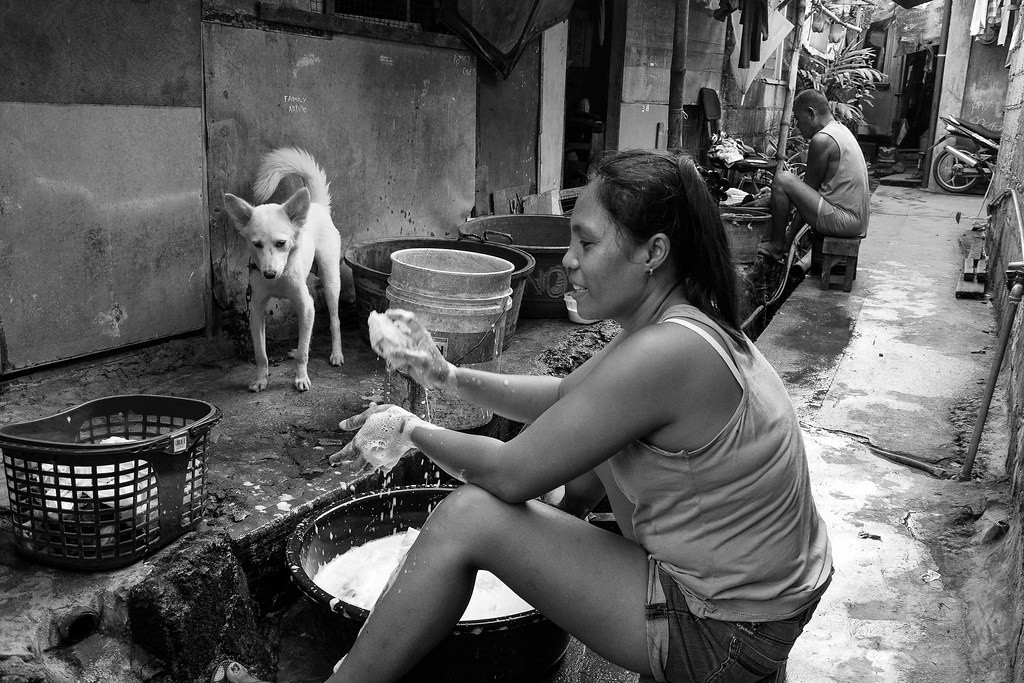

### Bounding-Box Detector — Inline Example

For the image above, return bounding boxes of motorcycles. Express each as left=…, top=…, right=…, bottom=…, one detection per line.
left=924, top=114, right=1003, bottom=194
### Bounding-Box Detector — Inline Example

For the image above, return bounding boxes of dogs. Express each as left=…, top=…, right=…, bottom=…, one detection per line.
left=223, top=146, right=344, bottom=392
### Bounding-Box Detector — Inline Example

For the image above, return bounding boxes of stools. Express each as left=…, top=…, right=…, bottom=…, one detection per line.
left=819, top=235, right=861, bottom=293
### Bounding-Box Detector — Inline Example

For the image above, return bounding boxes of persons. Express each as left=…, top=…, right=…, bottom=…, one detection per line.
left=211, top=148, right=834, bottom=683
left=769, top=89, right=870, bottom=247
